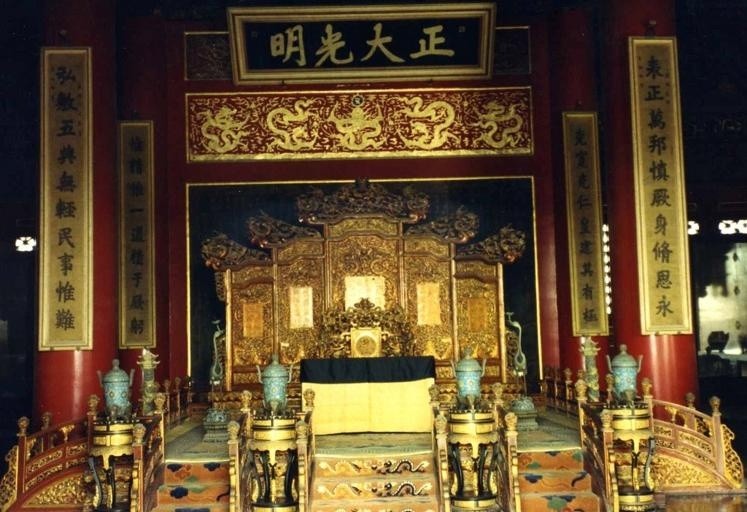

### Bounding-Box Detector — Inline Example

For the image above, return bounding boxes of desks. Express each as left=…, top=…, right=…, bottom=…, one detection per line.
left=300, top=356, right=436, bottom=436
